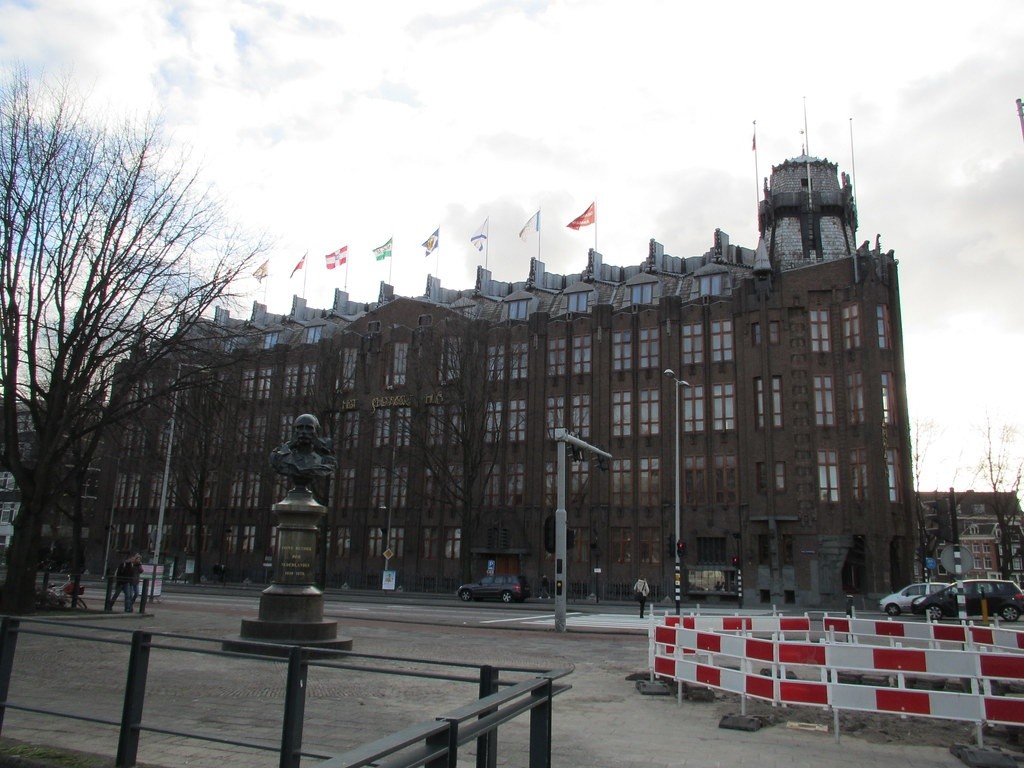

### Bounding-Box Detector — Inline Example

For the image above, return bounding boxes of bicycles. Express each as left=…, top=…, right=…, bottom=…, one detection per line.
left=48, top=574, right=90, bottom=609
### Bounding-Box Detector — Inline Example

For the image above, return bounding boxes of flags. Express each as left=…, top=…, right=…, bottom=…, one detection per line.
left=288, top=253, right=306, bottom=279
left=325, top=245, right=347, bottom=270
left=372, top=238, right=392, bottom=261
left=565, top=202, right=596, bottom=230
left=252, top=259, right=268, bottom=284
left=751, top=135, right=755, bottom=151
left=519, top=211, right=538, bottom=242
left=421, top=229, right=438, bottom=257
left=470, top=218, right=489, bottom=252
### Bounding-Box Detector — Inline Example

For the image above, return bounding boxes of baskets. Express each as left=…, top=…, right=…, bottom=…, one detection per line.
left=64, top=583, right=73, bottom=594
left=79, top=585, right=85, bottom=595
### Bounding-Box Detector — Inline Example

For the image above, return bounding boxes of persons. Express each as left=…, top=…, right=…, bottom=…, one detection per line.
left=109, top=553, right=144, bottom=612
left=537, top=574, right=551, bottom=600
left=633, top=574, right=650, bottom=618
left=212, top=560, right=229, bottom=586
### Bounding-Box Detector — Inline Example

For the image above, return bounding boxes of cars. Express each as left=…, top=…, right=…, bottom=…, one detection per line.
left=911, top=579, right=1024, bottom=622
left=879, top=582, right=958, bottom=616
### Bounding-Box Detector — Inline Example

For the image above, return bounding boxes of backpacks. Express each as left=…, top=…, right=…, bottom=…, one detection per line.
left=115, top=562, right=133, bottom=577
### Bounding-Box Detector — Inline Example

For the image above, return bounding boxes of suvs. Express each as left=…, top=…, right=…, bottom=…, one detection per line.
left=457, top=574, right=531, bottom=603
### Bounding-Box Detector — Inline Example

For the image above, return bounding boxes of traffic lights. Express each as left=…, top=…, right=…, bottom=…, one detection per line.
left=566, top=434, right=584, bottom=462
left=591, top=444, right=609, bottom=472
left=677, top=539, right=686, bottom=558
left=732, top=557, right=738, bottom=567
left=667, top=534, right=674, bottom=556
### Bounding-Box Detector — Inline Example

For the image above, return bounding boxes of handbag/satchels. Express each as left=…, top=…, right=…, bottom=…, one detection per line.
left=635, top=592, right=643, bottom=601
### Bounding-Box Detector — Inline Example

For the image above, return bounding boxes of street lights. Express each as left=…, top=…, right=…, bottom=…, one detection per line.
left=385, top=423, right=397, bottom=570
left=154, top=362, right=213, bottom=565
left=104, top=453, right=119, bottom=580
left=662, top=367, right=692, bottom=611
